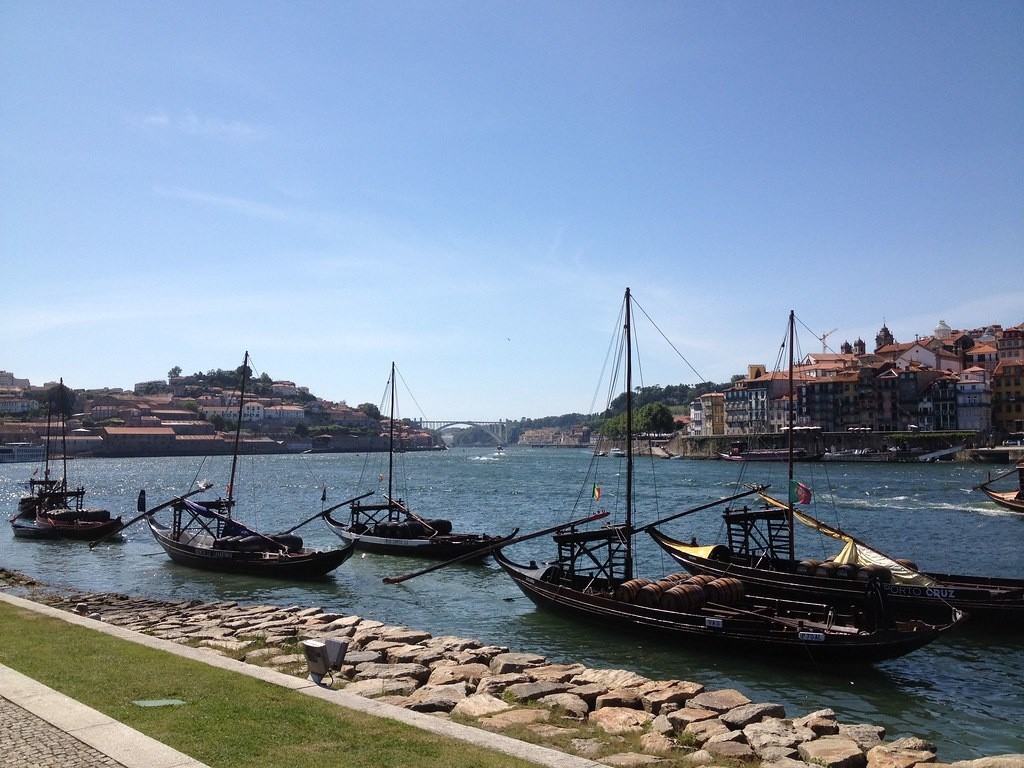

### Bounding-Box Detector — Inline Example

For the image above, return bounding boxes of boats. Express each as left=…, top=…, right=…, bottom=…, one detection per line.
left=977, top=454, right=1024, bottom=513
left=715, top=441, right=825, bottom=462
left=822, top=443, right=966, bottom=462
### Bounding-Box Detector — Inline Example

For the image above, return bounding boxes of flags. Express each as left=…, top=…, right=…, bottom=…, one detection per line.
left=790, top=479, right=812, bottom=505
left=592, top=484, right=600, bottom=500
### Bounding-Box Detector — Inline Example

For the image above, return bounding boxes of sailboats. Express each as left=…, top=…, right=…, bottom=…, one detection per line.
left=494, top=288, right=970, bottom=667
left=323, top=361, right=521, bottom=559
left=6, top=376, right=123, bottom=541
left=138, top=351, right=359, bottom=578
left=642, top=311, right=1024, bottom=642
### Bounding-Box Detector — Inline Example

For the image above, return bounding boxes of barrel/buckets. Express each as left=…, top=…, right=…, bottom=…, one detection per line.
left=797, top=556, right=918, bottom=583
left=213, top=534, right=304, bottom=553
left=614, top=573, right=744, bottom=612
left=45, top=508, right=110, bottom=521
left=374, top=518, right=452, bottom=538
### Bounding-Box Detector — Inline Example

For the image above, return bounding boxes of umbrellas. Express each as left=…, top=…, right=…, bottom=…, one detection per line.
left=780, top=426, right=822, bottom=432
